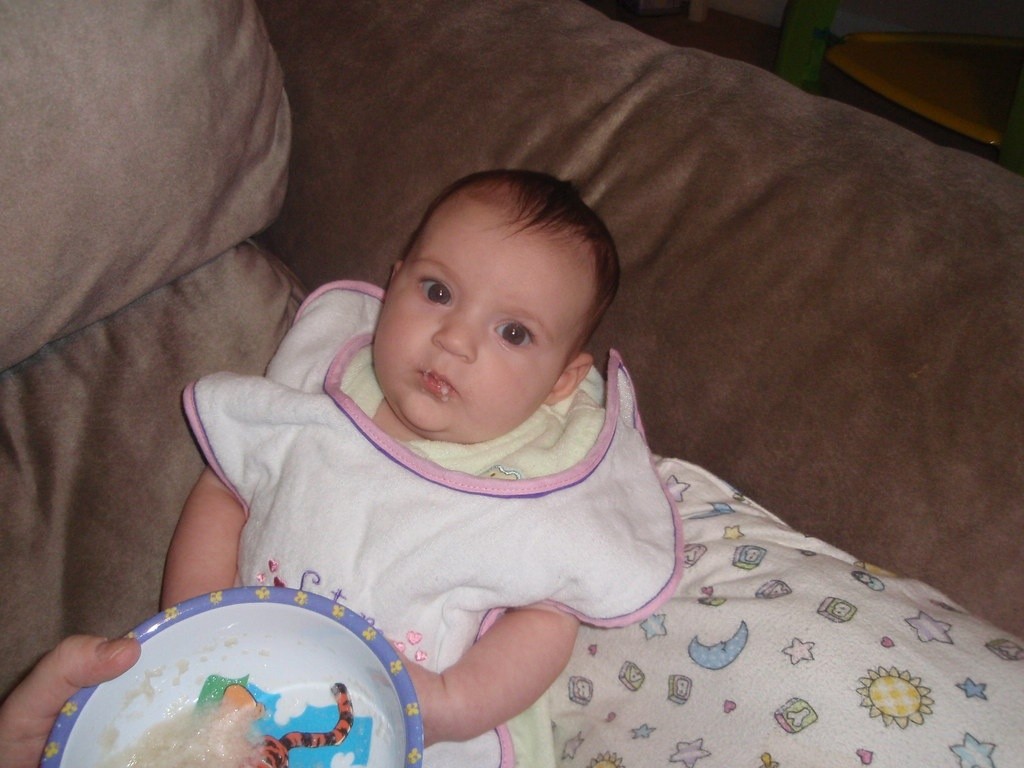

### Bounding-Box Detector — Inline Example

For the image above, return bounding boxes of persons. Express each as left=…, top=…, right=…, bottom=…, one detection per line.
left=0, top=634, right=142, bottom=768
left=161, top=167, right=685, bottom=768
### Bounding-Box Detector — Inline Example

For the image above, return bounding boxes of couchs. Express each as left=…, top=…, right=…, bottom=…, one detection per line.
left=0, top=0, right=1024, bottom=767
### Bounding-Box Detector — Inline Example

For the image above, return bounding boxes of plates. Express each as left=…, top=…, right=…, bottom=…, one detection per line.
left=39, top=584, right=423, bottom=768
